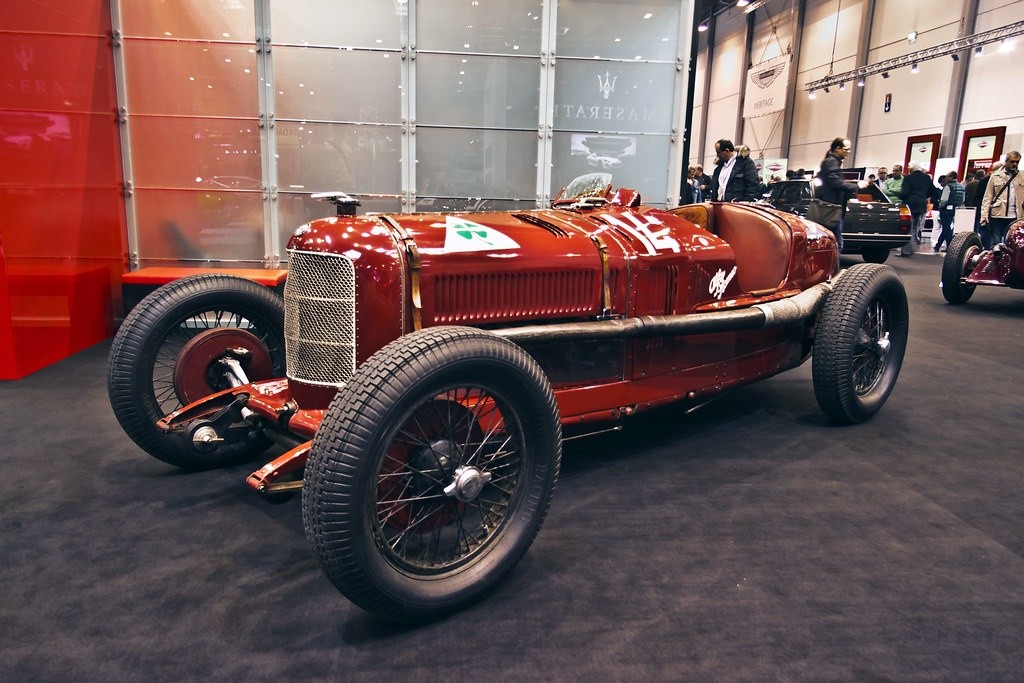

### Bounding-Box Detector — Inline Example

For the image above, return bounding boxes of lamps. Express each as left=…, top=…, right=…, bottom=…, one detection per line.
left=824, top=87, right=830, bottom=94
left=952, top=54, right=960, bottom=62
left=881, top=72, right=890, bottom=80
left=808, top=89, right=816, bottom=101
left=974, top=47, right=982, bottom=57
left=838, top=83, right=847, bottom=91
left=857, top=76, right=866, bottom=87
left=912, top=64, right=917, bottom=73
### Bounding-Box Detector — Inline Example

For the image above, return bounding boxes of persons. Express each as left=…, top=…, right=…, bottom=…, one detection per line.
left=759, top=169, right=804, bottom=194
left=868, top=174, right=875, bottom=182
left=915, top=161, right=1003, bottom=253
left=741, top=146, right=750, bottom=157
left=883, top=165, right=904, bottom=203
left=813, top=137, right=869, bottom=254
left=980, top=151, right=1024, bottom=245
left=687, top=167, right=702, bottom=204
left=875, top=167, right=888, bottom=190
left=895, top=161, right=934, bottom=258
left=711, top=139, right=760, bottom=202
left=695, top=165, right=712, bottom=202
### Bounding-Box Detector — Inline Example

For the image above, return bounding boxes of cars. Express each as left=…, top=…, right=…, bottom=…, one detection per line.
left=761, top=179, right=913, bottom=264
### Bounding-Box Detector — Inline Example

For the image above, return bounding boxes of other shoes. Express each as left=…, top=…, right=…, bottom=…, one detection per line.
left=894, top=253, right=911, bottom=258
left=934, top=246, right=939, bottom=252
left=941, top=249, right=947, bottom=252
left=916, top=237, right=926, bottom=244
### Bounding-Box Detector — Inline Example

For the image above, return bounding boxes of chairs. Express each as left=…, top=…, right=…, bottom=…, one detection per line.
left=667, top=203, right=791, bottom=294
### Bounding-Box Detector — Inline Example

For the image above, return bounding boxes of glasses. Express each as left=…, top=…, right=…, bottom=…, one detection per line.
left=880, top=172, right=887, bottom=174
left=1010, top=161, right=1019, bottom=163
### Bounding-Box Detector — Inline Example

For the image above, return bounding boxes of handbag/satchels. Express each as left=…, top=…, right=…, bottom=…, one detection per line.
left=806, top=199, right=842, bottom=229
